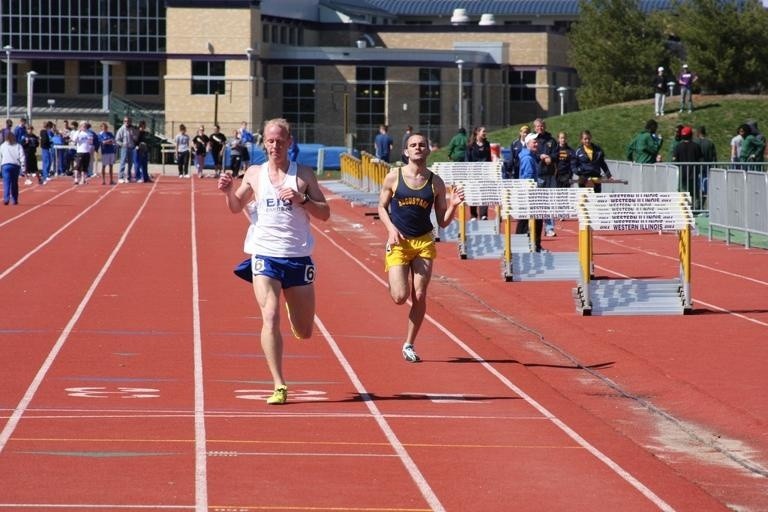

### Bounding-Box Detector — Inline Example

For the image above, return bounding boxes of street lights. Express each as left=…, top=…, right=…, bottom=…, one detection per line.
left=26, top=70, right=39, bottom=130
left=3, top=44, right=13, bottom=122
left=244, top=47, right=253, bottom=129
left=556, top=86, right=568, bottom=115
left=667, top=81, right=676, bottom=96
left=455, top=59, right=465, bottom=130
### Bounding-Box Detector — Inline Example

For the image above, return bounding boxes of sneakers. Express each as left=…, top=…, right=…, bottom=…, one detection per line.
left=545, top=229, right=556, bottom=237
left=469, top=217, right=477, bottom=222
left=400, top=342, right=423, bottom=363
left=267, top=384, right=289, bottom=406
left=481, top=216, right=487, bottom=220
left=117, top=178, right=130, bottom=183
left=73, top=179, right=87, bottom=185
left=655, top=113, right=660, bottom=117
left=687, top=110, right=692, bottom=114
left=661, top=112, right=664, bottom=116
left=285, top=302, right=305, bottom=340
left=179, top=174, right=191, bottom=178
left=101, top=180, right=116, bottom=185
left=25, top=176, right=54, bottom=186
left=678, top=109, right=684, bottom=113
left=135, top=178, right=152, bottom=183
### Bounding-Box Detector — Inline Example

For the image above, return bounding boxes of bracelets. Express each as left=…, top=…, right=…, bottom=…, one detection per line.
left=299, top=193, right=310, bottom=205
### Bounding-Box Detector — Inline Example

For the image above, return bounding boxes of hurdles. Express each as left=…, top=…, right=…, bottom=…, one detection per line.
left=500, top=187, right=596, bottom=279
left=502, top=211, right=582, bottom=281
left=576, top=222, right=696, bottom=316
left=571, top=191, right=695, bottom=308
left=459, top=200, right=536, bottom=260
left=430, top=162, right=539, bottom=256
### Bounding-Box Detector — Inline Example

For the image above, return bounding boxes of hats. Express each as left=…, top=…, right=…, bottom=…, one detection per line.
left=525, top=132, right=540, bottom=145
left=519, top=125, right=531, bottom=131
left=682, top=64, right=688, bottom=69
left=681, top=127, right=693, bottom=136
left=658, top=66, right=665, bottom=72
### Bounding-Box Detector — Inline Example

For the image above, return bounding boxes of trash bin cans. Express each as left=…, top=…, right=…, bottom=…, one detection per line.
left=490, top=143, right=500, bottom=161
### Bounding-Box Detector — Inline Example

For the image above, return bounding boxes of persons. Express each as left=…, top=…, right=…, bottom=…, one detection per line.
left=376, top=131, right=467, bottom=364
left=372, top=122, right=394, bottom=164
left=216, top=115, right=331, bottom=407
left=510, top=117, right=767, bottom=254
left=649, top=65, right=667, bottom=117
left=447, top=127, right=468, bottom=163
left=401, top=125, right=414, bottom=163
left=676, top=63, right=698, bottom=115
left=0, top=114, right=252, bottom=186
left=0, top=131, right=27, bottom=205
left=462, top=126, right=490, bottom=221
